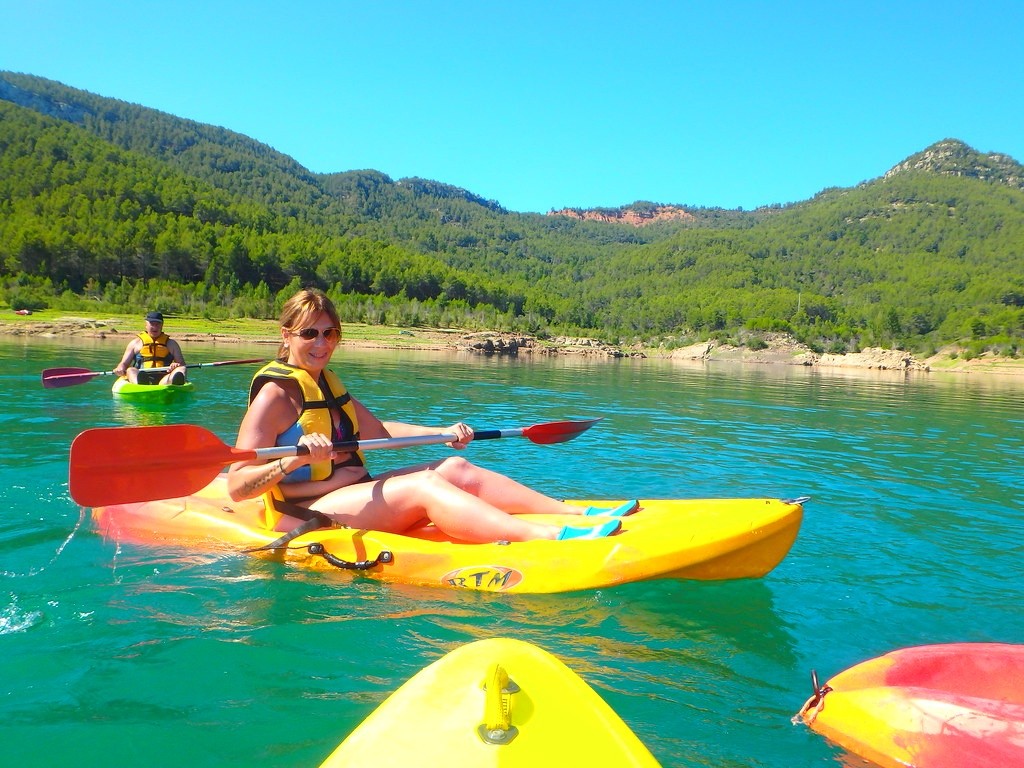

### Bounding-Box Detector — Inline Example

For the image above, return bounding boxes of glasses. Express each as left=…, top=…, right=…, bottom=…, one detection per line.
left=291, top=328, right=341, bottom=342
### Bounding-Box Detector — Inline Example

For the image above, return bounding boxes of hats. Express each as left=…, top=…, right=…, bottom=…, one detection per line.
left=144, top=311, right=163, bottom=322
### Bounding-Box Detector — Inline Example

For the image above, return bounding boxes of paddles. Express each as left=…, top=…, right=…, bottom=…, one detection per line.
left=67, top=417, right=608, bottom=508
left=41, top=357, right=268, bottom=390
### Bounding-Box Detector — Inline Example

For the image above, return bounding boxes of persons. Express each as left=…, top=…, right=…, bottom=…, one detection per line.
left=225, top=286, right=641, bottom=545
left=113, top=309, right=188, bottom=387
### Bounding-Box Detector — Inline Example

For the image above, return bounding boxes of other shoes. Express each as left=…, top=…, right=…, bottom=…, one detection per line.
left=556, top=520, right=622, bottom=541
left=172, top=371, right=184, bottom=386
left=585, top=500, right=639, bottom=516
left=137, top=370, right=149, bottom=385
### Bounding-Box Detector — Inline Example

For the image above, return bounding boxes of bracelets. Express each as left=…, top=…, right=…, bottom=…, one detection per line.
left=279, top=459, right=289, bottom=477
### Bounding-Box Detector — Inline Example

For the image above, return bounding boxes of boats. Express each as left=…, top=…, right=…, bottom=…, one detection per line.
left=789, top=641, right=1024, bottom=768
left=111, top=375, right=194, bottom=395
left=87, top=467, right=808, bottom=596
